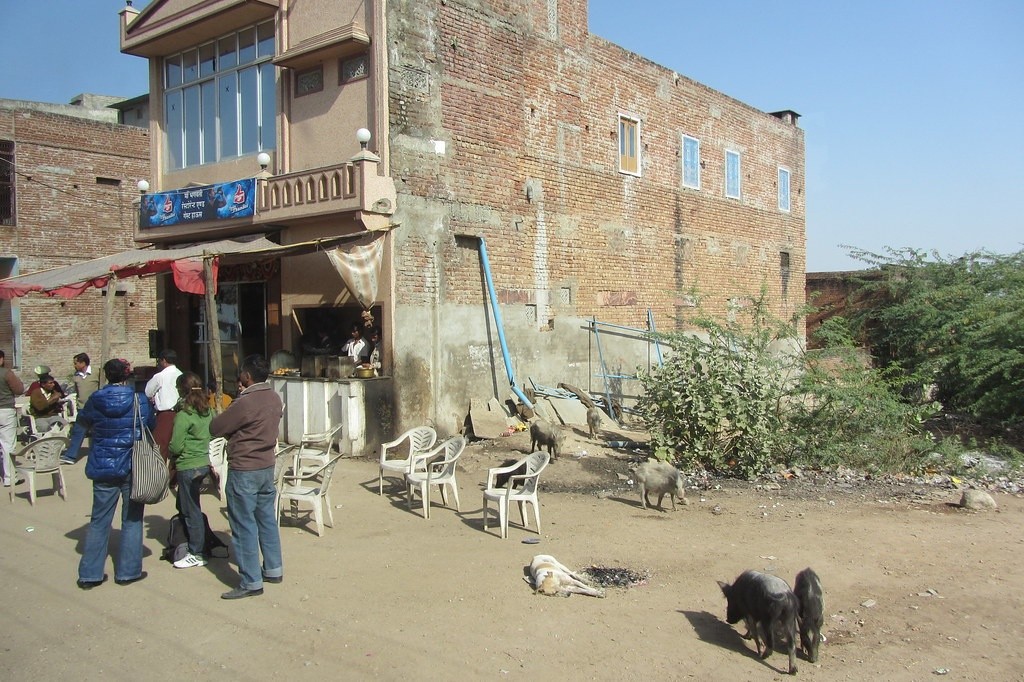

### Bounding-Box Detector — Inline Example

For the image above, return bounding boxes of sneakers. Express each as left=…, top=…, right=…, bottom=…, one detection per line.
left=174, top=553, right=208, bottom=569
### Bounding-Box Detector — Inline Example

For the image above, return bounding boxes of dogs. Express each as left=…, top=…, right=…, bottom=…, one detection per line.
left=529, top=555, right=605, bottom=598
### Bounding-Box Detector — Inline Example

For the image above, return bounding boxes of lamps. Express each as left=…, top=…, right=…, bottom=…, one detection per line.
left=357, top=129, right=370, bottom=142
left=258, top=154, right=271, bottom=167
left=138, top=180, right=150, bottom=191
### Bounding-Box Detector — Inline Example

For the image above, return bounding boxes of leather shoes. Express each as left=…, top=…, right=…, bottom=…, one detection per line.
left=262, top=572, right=283, bottom=583
left=222, top=585, right=263, bottom=599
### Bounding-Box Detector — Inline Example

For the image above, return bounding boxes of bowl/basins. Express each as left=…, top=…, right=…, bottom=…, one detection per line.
left=357, top=368, right=374, bottom=377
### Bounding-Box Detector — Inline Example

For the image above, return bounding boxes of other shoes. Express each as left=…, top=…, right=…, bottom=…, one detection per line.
left=60, top=455, right=75, bottom=465
left=4, top=479, right=26, bottom=487
left=115, top=572, right=148, bottom=587
left=82, top=574, right=108, bottom=591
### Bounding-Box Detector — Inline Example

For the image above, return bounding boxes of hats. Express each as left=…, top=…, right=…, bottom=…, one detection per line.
left=35, top=365, right=51, bottom=375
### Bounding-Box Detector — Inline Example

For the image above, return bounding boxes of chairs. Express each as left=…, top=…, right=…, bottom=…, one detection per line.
left=482, top=451, right=549, bottom=540
left=378, top=426, right=437, bottom=506
left=209, top=422, right=344, bottom=537
left=405, top=436, right=466, bottom=519
left=7, top=395, right=76, bottom=506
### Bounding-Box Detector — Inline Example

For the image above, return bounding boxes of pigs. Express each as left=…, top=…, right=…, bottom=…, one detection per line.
left=716, top=568, right=825, bottom=676
left=529, top=419, right=567, bottom=462
left=635, top=461, right=685, bottom=511
left=586, top=408, right=602, bottom=440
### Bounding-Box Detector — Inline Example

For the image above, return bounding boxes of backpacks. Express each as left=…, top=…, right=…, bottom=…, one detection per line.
left=163, top=513, right=228, bottom=564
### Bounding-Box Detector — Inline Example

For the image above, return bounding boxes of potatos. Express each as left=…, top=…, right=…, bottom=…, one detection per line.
left=272, top=367, right=290, bottom=374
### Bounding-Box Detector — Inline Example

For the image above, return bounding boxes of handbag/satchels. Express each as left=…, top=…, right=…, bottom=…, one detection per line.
left=130, top=392, right=169, bottom=504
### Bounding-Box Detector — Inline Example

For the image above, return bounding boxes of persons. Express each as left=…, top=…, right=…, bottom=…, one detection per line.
left=204, top=187, right=228, bottom=221
left=139, top=194, right=157, bottom=227
left=167, top=371, right=216, bottom=568
left=143, top=348, right=246, bottom=487
left=0, top=350, right=26, bottom=488
left=75, top=358, right=156, bottom=588
left=312, top=322, right=384, bottom=378
left=208, top=355, right=283, bottom=600
left=27, top=353, right=107, bottom=465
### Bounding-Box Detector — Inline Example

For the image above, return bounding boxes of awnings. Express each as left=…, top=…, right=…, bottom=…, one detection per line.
left=0, top=225, right=405, bottom=303
